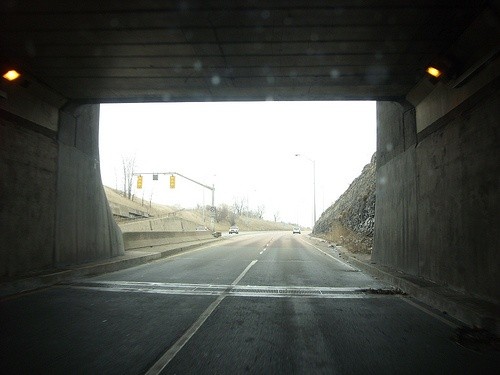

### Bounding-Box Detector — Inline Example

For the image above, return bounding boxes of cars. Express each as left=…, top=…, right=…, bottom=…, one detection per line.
left=229, top=226, right=239, bottom=234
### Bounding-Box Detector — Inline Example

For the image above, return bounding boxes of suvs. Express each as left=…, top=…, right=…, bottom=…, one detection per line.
left=293, top=227, right=300, bottom=234
